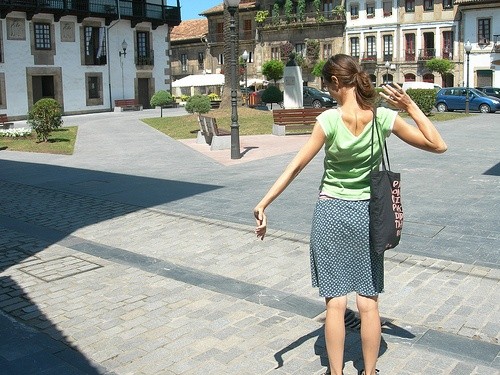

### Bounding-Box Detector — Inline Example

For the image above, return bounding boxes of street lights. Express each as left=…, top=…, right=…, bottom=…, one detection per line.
left=464, top=40, right=472, bottom=115
left=385, top=61, right=390, bottom=85
left=242, top=49, right=249, bottom=95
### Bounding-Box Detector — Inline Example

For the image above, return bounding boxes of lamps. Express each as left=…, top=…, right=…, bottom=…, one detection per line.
left=119, top=39, right=128, bottom=58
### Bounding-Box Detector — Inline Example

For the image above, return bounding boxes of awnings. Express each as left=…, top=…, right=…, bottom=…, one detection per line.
left=247, top=78, right=270, bottom=91
left=172, top=74, right=225, bottom=87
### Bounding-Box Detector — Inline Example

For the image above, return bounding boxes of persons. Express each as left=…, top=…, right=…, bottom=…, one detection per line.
left=254, top=54, right=447, bottom=375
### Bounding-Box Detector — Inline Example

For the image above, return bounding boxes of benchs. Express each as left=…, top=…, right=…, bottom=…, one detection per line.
left=114, top=99, right=143, bottom=112
left=0, top=114, right=14, bottom=129
left=273, top=108, right=328, bottom=136
left=196, top=115, right=231, bottom=151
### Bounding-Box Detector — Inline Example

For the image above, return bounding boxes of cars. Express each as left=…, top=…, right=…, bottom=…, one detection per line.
left=304, top=85, right=338, bottom=109
left=433, top=86, right=500, bottom=114
left=463, top=86, right=500, bottom=99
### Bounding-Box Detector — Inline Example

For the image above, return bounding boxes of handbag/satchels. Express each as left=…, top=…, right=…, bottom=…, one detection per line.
left=371, top=107, right=404, bottom=256
